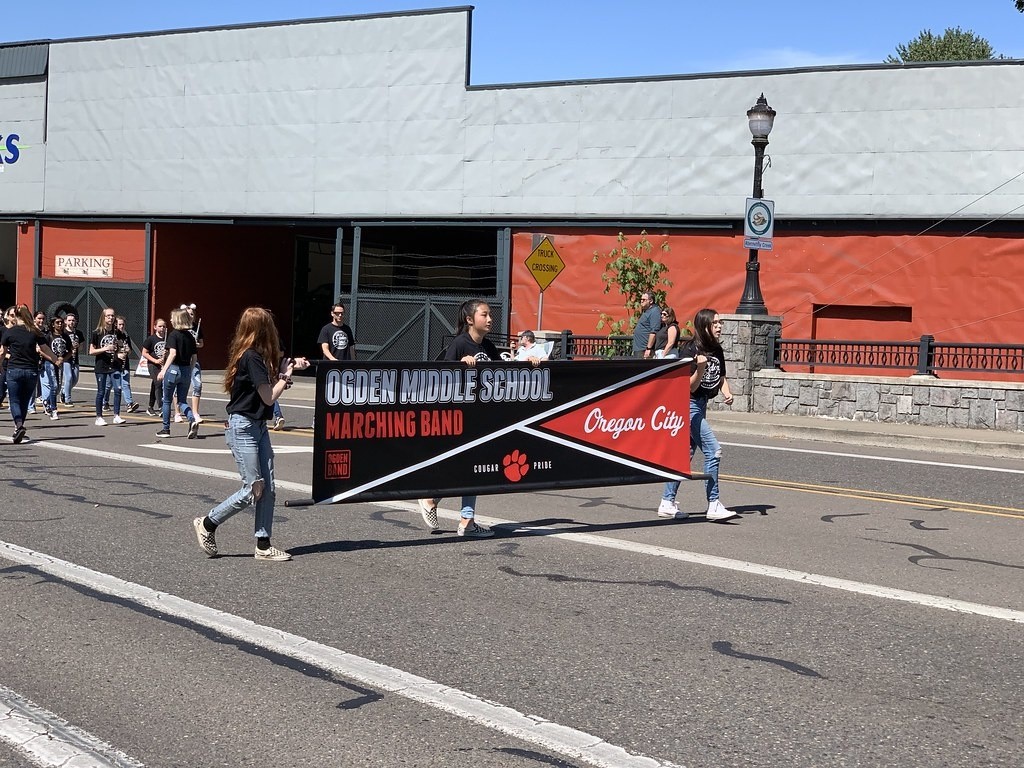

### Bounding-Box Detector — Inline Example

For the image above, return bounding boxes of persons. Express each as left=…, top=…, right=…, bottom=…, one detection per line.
left=0, top=303, right=59, bottom=444
left=0, top=306, right=85, bottom=420
left=89, top=308, right=139, bottom=426
left=418, top=298, right=495, bottom=537
left=142, top=304, right=204, bottom=439
left=633, top=292, right=680, bottom=359
left=658, top=309, right=737, bottom=520
left=193, top=308, right=310, bottom=561
left=317, top=303, right=357, bottom=360
left=510, top=330, right=546, bottom=368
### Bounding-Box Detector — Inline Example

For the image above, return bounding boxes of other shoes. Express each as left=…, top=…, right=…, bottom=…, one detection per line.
left=30, top=410, right=38, bottom=414
left=194, top=414, right=203, bottom=423
left=0, top=404, right=5, bottom=409
left=175, top=413, right=183, bottom=423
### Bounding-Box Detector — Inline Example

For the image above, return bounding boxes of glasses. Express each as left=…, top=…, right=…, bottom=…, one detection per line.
left=105, top=315, right=115, bottom=318
left=9, top=314, right=14, bottom=317
left=711, top=320, right=724, bottom=327
left=661, top=313, right=667, bottom=316
left=333, top=312, right=345, bottom=316
left=640, top=299, right=651, bottom=302
left=56, top=321, right=64, bottom=324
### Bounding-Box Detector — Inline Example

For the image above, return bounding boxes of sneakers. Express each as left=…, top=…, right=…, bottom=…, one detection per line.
left=13, top=426, right=30, bottom=444
left=657, top=499, right=689, bottom=519
left=64, top=403, right=74, bottom=408
left=113, top=415, right=126, bottom=424
left=458, top=522, right=495, bottom=537
left=187, top=421, right=199, bottom=439
left=706, top=500, right=737, bottom=520
left=126, top=403, right=139, bottom=413
left=60, top=389, right=65, bottom=404
left=95, top=418, right=107, bottom=426
left=155, top=429, right=170, bottom=438
left=43, top=400, right=58, bottom=420
left=418, top=499, right=439, bottom=529
left=102, top=406, right=111, bottom=411
left=146, top=407, right=156, bottom=416
left=193, top=517, right=219, bottom=557
left=273, top=415, right=285, bottom=431
left=254, top=546, right=291, bottom=561
left=159, top=409, right=163, bottom=417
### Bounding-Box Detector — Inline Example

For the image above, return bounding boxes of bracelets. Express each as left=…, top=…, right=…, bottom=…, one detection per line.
left=279, top=373, right=293, bottom=389
left=646, top=347, right=651, bottom=350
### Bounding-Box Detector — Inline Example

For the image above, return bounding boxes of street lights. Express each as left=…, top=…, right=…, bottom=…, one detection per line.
left=735, top=92, right=777, bottom=316
